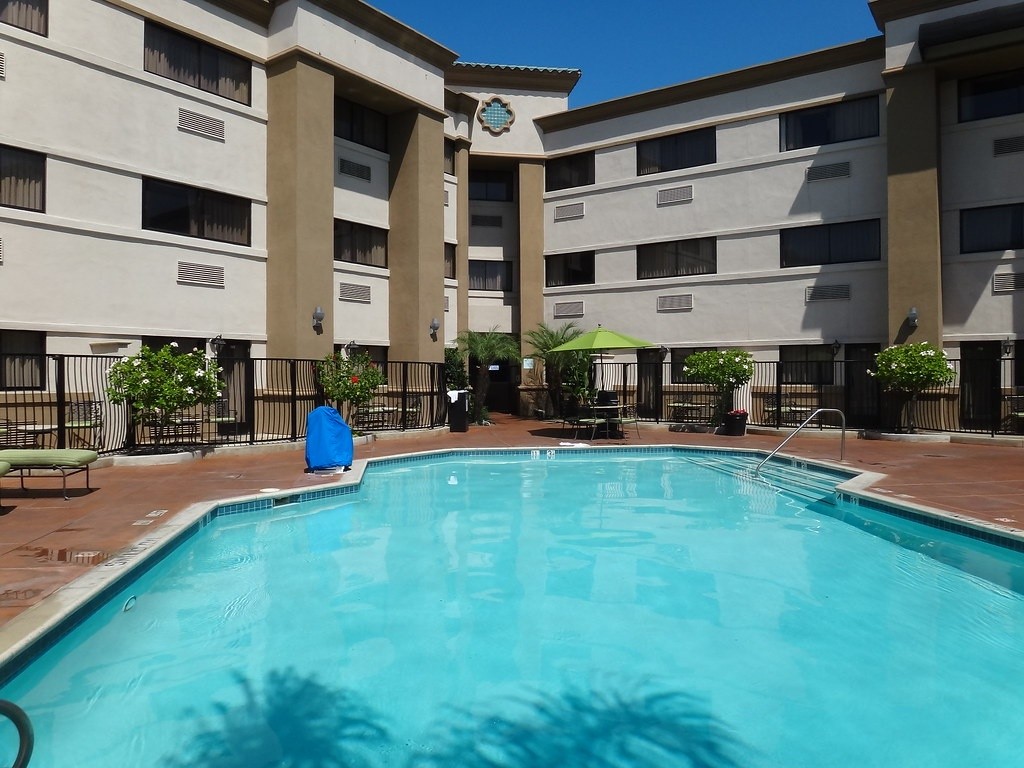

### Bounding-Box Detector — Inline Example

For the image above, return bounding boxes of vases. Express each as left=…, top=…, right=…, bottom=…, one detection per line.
left=725, top=413, right=748, bottom=436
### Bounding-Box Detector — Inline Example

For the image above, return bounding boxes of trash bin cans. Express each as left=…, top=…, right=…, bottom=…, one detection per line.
left=727, top=413, right=748, bottom=436
left=447, top=391, right=469, bottom=432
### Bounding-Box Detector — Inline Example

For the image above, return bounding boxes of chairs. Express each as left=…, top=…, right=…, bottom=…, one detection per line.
left=0, top=448, right=98, bottom=500
left=358, top=396, right=384, bottom=429
left=763, top=393, right=791, bottom=426
left=608, top=402, right=642, bottom=440
left=667, top=392, right=693, bottom=422
left=66, top=400, right=104, bottom=451
left=204, top=398, right=238, bottom=442
left=564, top=399, right=587, bottom=431
left=576, top=403, right=607, bottom=440
left=141, top=408, right=181, bottom=444
left=397, top=393, right=423, bottom=428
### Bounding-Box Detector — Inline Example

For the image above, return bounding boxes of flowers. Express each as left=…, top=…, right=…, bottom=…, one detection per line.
left=726, top=408, right=746, bottom=415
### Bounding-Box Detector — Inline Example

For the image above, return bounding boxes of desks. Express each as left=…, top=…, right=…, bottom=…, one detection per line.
left=16, top=424, right=58, bottom=449
left=179, top=418, right=203, bottom=443
left=374, top=407, right=398, bottom=429
left=588, top=405, right=627, bottom=442
left=682, top=405, right=707, bottom=423
left=790, top=407, right=812, bottom=427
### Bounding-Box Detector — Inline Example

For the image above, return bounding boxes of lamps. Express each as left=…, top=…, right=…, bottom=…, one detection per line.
left=830, top=339, right=841, bottom=356
left=346, top=340, right=360, bottom=357
left=659, top=345, right=670, bottom=359
left=428, top=317, right=440, bottom=336
left=313, top=306, right=325, bottom=327
left=906, top=306, right=919, bottom=328
left=1002, top=337, right=1014, bottom=356
left=209, top=335, right=227, bottom=356
